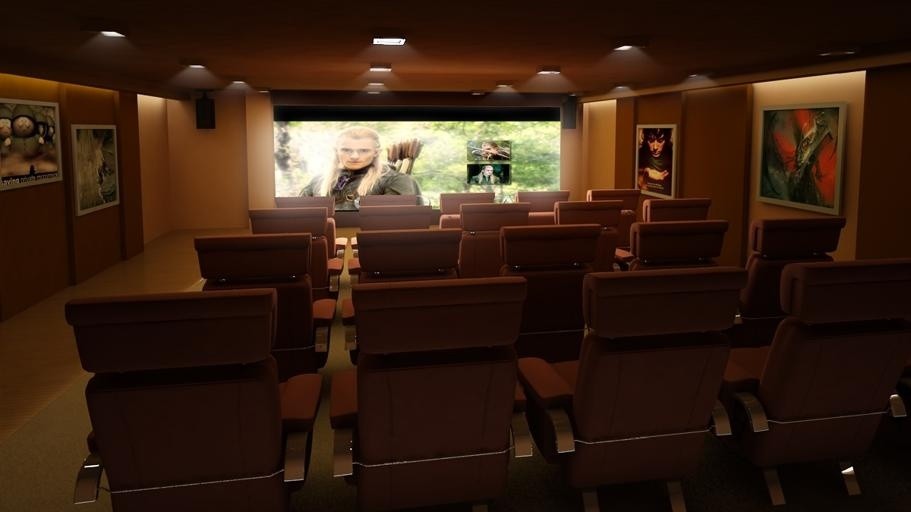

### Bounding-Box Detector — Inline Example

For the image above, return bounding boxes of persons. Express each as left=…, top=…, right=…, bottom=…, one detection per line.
left=99, top=135, right=117, bottom=203
left=639, top=129, right=673, bottom=195
left=472, top=142, right=508, bottom=160
left=298, top=126, right=424, bottom=212
left=471, top=165, right=500, bottom=184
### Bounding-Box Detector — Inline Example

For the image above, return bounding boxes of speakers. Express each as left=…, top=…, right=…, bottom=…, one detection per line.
left=196, top=98, right=215, bottom=128
left=560, top=96, right=576, bottom=128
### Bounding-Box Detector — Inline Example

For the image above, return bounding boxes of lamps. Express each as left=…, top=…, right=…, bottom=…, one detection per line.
left=369, top=62, right=393, bottom=73
left=496, top=79, right=514, bottom=90
left=536, top=64, right=561, bottom=75
left=370, top=28, right=407, bottom=46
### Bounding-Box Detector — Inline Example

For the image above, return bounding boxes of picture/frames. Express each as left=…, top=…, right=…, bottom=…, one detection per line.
left=753, top=103, right=850, bottom=217
left=70, top=123, right=122, bottom=217
left=634, top=122, right=681, bottom=201
left=0, top=97, right=65, bottom=192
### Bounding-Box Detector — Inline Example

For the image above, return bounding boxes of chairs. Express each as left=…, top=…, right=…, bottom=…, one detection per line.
left=327, top=272, right=530, bottom=511
left=716, top=258, right=910, bottom=510
left=192, top=190, right=852, bottom=383
left=515, top=263, right=750, bottom=512
left=61, top=286, right=326, bottom=511
